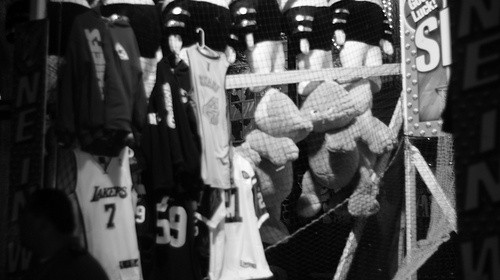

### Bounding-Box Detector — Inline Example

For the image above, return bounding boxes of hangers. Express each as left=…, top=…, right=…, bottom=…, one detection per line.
left=195, top=26, right=219, bottom=58
